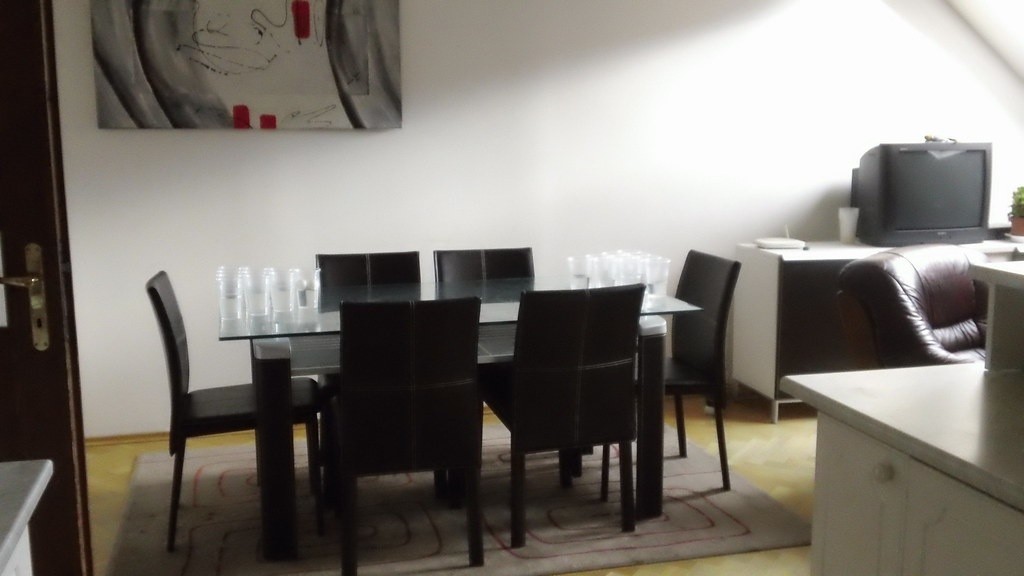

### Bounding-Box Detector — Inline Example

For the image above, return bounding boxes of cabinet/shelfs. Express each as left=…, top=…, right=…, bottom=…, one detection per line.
left=731, top=241, right=1024, bottom=576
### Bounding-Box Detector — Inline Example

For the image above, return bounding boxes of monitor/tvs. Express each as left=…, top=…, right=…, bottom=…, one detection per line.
left=851, top=142, right=992, bottom=247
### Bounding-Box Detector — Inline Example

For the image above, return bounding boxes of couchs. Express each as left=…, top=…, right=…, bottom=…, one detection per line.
left=839, top=243, right=985, bottom=368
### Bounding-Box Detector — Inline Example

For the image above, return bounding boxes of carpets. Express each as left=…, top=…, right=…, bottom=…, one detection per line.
left=106, top=420, right=811, bottom=576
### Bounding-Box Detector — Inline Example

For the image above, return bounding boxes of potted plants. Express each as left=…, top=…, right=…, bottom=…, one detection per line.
left=1008, top=186, right=1024, bottom=238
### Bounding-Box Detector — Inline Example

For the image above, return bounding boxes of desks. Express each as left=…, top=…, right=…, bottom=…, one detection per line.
left=218, top=272, right=705, bottom=560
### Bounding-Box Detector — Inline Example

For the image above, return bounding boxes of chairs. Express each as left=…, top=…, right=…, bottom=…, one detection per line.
left=317, top=294, right=484, bottom=576
left=147, top=272, right=326, bottom=551
left=481, top=283, right=648, bottom=549
left=600, top=250, right=740, bottom=505
left=317, top=247, right=535, bottom=286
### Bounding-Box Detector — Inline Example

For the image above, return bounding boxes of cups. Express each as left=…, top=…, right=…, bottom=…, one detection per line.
left=569, top=250, right=672, bottom=297
left=839, top=207, right=859, bottom=244
left=216, top=266, right=320, bottom=315
